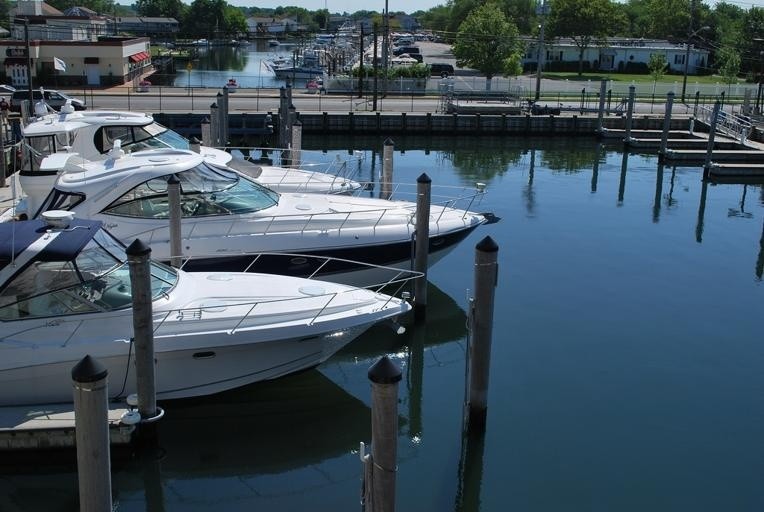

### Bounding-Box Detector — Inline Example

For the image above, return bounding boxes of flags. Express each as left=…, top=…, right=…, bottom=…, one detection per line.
left=54, top=57, right=66, bottom=72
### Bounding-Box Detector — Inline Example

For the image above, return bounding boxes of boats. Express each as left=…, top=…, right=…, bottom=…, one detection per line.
left=105, top=365, right=411, bottom=479
left=264, top=20, right=374, bottom=80
left=3, top=108, right=361, bottom=226
left=0, top=209, right=426, bottom=406
left=22, top=144, right=496, bottom=288
left=153, top=38, right=254, bottom=49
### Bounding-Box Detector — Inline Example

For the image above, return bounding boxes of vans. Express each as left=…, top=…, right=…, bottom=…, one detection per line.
left=10, top=89, right=88, bottom=115
left=388, top=30, right=455, bottom=79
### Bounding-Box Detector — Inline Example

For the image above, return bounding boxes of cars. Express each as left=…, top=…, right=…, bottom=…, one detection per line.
left=0, top=84, right=17, bottom=97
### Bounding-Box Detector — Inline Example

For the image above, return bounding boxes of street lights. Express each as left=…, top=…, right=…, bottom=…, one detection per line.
left=113, top=1, right=121, bottom=35
left=681, top=25, right=711, bottom=101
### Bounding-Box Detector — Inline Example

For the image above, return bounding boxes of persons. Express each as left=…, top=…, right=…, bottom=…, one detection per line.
left=0, top=96, right=10, bottom=125
